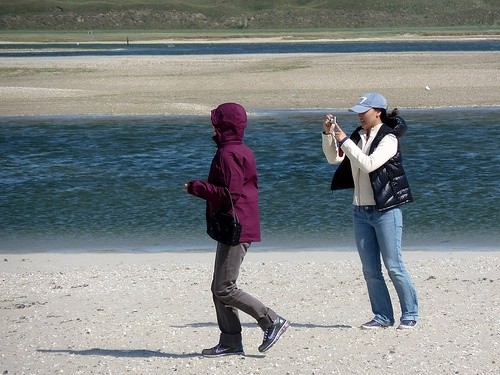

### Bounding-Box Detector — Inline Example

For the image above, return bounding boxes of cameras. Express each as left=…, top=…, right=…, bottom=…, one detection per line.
left=329, top=116, right=336, bottom=124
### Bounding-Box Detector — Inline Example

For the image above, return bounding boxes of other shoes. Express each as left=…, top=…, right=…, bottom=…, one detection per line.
left=398, top=320, right=418, bottom=330
left=361, top=318, right=393, bottom=330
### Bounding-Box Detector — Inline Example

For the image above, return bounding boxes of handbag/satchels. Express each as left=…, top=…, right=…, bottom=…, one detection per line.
left=206, top=186, right=243, bottom=246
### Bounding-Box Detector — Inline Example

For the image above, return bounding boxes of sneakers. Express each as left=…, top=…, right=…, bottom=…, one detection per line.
left=258, top=314, right=290, bottom=353
left=201, top=341, right=246, bottom=358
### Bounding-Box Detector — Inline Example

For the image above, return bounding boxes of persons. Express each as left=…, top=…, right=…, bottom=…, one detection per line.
left=184, top=102, right=290, bottom=358
left=321, top=92, right=419, bottom=331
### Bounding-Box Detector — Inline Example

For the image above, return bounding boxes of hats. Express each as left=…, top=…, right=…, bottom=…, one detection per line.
left=347, top=91, right=388, bottom=114
